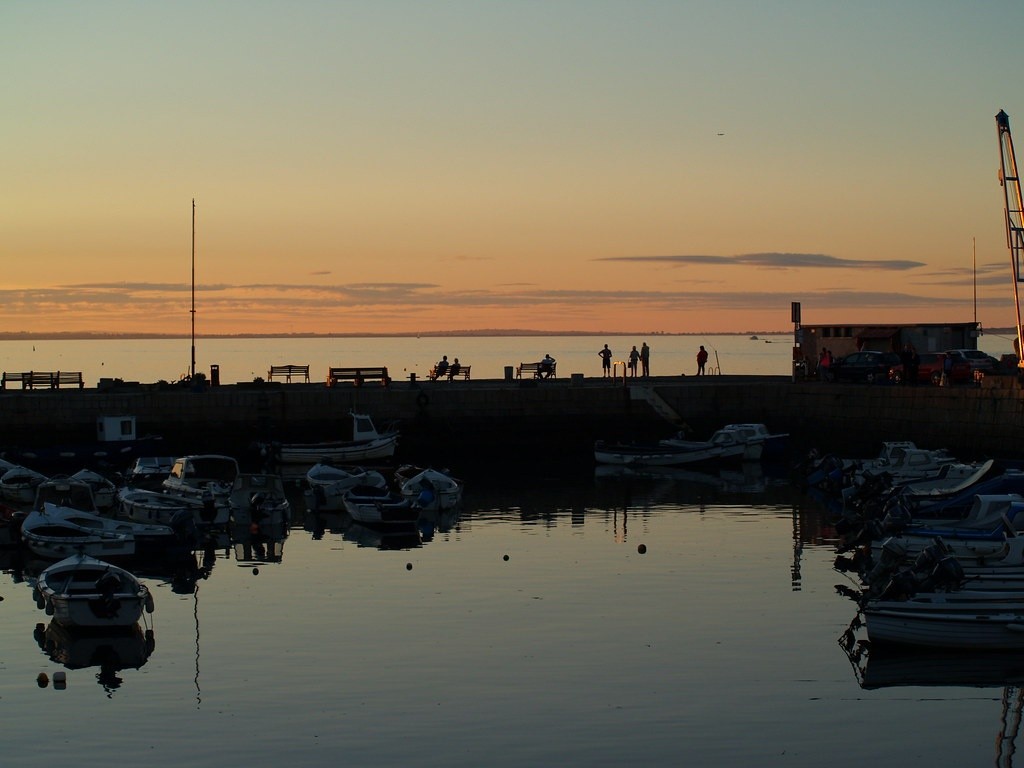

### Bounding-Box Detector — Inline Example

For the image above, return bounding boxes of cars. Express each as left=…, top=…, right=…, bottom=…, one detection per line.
left=825, top=349, right=1003, bottom=388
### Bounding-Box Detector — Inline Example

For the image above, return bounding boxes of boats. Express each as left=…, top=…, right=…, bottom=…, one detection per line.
left=343, top=483, right=423, bottom=527
left=32, top=474, right=100, bottom=516
left=125, top=457, right=180, bottom=495
left=37, top=549, right=149, bottom=630
left=857, top=645, right=1023, bottom=692
left=306, top=463, right=355, bottom=488
left=162, top=454, right=292, bottom=526
left=20, top=509, right=136, bottom=567
left=594, top=428, right=749, bottom=472
left=394, top=463, right=461, bottom=512
left=280, top=413, right=400, bottom=465
left=304, top=470, right=390, bottom=511
left=805, top=441, right=1024, bottom=651
left=40, top=616, right=148, bottom=699
left=658, top=423, right=790, bottom=463
left=43, top=501, right=176, bottom=553
left=115, top=471, right=230, bottom=539
left=17, top=413, right=164, bottom=470
left=0, top=464, right=52, bottom=505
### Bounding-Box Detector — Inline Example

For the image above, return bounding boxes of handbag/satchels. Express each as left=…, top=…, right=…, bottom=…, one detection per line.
left=628, top=357, right=632, bottom=368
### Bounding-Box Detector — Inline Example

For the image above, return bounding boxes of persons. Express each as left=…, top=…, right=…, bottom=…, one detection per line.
left=629, top=345, right=641, bottom=377
left=448, top=358, right=461, bottom=384
left=696, top=345, right=708, bottom=377
left=815, top=347, right=835, bottom=384
left=900, top=346, right=921, bottom=388
left=598, top=344, right=613, bottom=378
left=537, top=354, right=556, bottom=379
left=432, top=355, right=449, bottom=383
left=641, top=342, right=650, bottom=378
left=943, top=354, right=954, bottom=388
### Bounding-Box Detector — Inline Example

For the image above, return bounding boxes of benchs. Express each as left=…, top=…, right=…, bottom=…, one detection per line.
left=516, top=362, right=559, bottom=379
left=326, top=367, right=392, bottom=387
left=21, top=371, right=86, bottom=389
left=1, top=372, right=55, bottom=391
left=430, top=365, right=471, bottom=382
left=267, top=364, right=310, bottom=384
